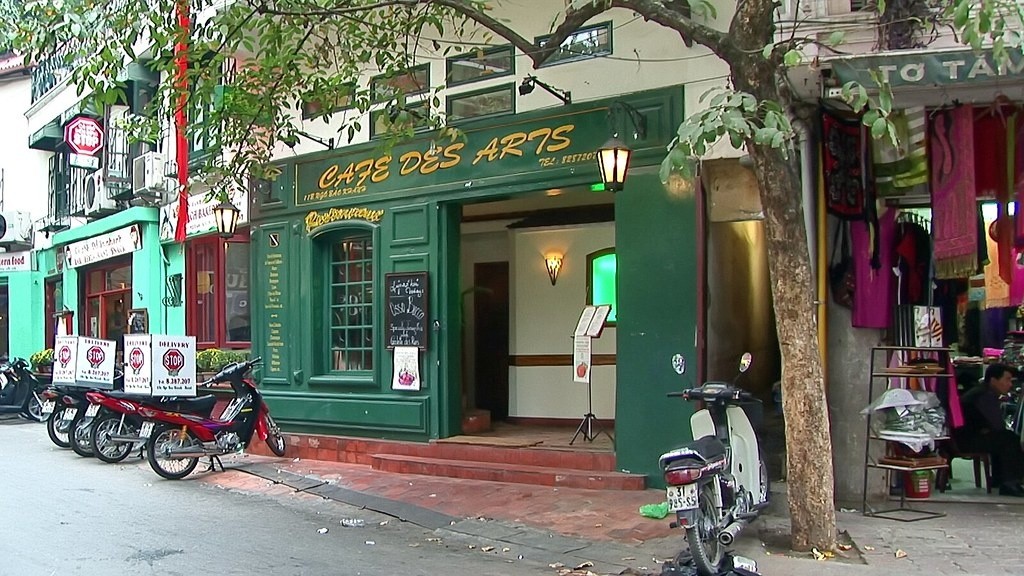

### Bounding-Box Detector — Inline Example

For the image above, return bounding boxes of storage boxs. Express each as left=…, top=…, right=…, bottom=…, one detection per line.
left=53, top=334, right=116, bottom=390
left=123, top=334, right=198, bottom=397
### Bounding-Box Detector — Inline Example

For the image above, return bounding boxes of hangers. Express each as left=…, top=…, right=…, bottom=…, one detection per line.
left=897, top=212, right=933, bottom=235
left=980, top=90, right=1019, bottom=121
left=929, top=93, right=955, bottom=121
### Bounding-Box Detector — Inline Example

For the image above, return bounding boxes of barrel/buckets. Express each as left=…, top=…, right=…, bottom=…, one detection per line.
left=905, top=471, right=931, bottom=497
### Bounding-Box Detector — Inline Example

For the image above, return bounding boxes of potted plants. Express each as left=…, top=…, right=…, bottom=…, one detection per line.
left=30, top=348, right=53, bottom=372
left=196, top=349, right=250, bottom=382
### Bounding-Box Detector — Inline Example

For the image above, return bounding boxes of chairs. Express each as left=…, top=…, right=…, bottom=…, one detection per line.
left=938, top=409, right=992, bottom=492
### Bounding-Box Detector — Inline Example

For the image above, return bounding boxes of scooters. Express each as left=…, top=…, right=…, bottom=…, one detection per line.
left=0, top=354, right=53, bottom=422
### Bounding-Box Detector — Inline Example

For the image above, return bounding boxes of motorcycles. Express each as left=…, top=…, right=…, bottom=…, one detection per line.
left=656, top=350, right=767, bottom=575
left=42, top=356, right=287, bottom=480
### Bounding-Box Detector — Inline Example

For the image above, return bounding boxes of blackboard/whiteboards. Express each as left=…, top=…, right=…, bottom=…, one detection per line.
left=127, top=308, right=148, bottom=335
left=384, top=271, right=429, bottom=352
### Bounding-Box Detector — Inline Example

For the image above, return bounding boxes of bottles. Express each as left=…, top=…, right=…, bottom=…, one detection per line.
left=66, top=246, right=71, bottom=265
left=130, top=226, right=138, bottom=249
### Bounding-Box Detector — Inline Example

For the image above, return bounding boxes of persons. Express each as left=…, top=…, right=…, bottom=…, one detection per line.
left=949, top=362, right=1024, bottom=498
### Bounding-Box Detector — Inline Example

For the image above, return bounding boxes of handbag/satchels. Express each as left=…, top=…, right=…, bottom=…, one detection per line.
left=828, top=260, right=856, bottom=309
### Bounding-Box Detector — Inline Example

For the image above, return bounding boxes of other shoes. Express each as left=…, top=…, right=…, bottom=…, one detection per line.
left=999, top=485, right=1024, bottom=496
left=990, top=478, right=999, bottom=488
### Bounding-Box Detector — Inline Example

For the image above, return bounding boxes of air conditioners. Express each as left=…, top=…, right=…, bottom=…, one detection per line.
left=134, top=152, right=161, bottom=192
left=0, top=211, right=30, bottom=246
left=84, top=168, right=123, bottom=217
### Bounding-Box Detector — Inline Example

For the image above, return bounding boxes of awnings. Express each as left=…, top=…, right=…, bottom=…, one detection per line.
left=112, top=58, right=160, bottom=85
left=29, top=117, right=64, bottom=153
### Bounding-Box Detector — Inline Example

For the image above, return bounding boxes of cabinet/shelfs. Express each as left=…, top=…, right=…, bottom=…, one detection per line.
left=863, top=345, right=956, bottom=522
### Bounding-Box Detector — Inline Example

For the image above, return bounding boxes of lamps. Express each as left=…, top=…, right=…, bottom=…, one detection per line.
left=212, top=172, right=270, bottom=240
left=544, top=258, right=563, bottom=286
left=519, top=77, right=572, bottom=105
left=286, top=130, right=335, bottom=150
left=390, top=106, right=431, bottom=126
left=595, top=98, right=648, bottom=192
